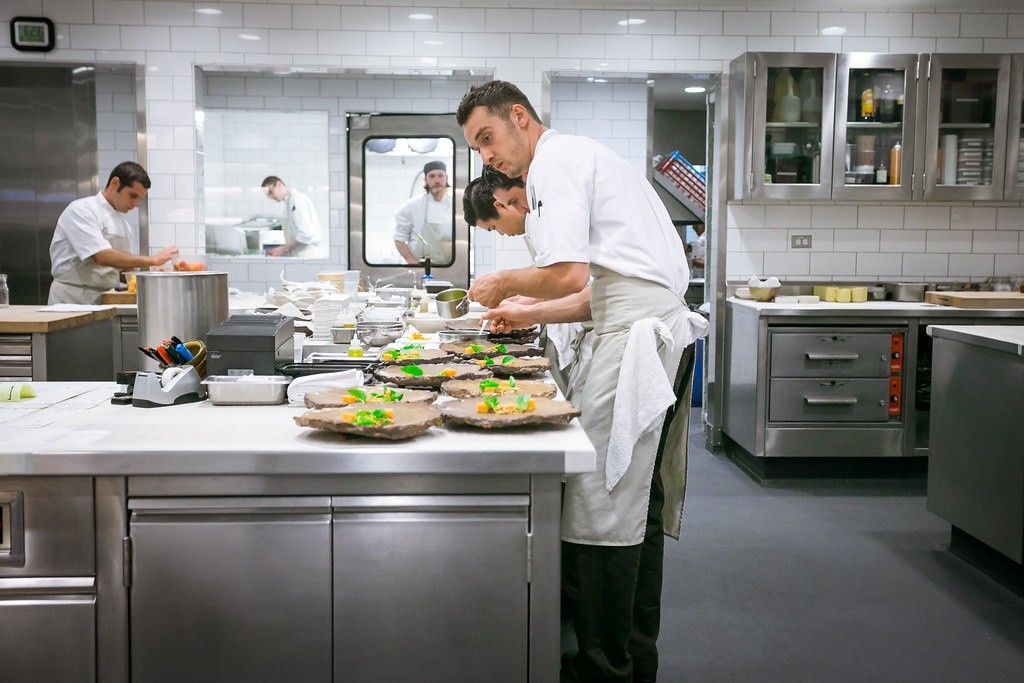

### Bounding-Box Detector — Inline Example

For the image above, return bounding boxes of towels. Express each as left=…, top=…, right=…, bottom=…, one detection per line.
left=605, top=318, right=678, bottom=497
left=286, top=368, right=365, bottom=407
left=546, top=323, right=586, bottom=372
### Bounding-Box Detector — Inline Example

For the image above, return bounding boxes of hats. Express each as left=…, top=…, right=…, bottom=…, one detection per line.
left=424, top=161, right=446, bottom=175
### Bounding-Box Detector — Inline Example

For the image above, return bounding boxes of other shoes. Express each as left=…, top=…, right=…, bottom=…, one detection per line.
left=561, top=650, right=656, bottom=683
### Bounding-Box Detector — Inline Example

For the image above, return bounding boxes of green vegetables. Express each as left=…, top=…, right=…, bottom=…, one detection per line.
left=349, top=343, right=529, bottom=429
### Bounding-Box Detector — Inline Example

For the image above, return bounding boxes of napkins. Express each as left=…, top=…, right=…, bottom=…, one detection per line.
left=725, top=47, right=1024, bottom=206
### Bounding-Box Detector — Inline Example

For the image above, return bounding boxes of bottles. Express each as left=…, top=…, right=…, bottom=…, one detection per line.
left=0, top=274, right=9, bottom=307
left=771, top=68, right=822, bottom=121
left=890, top=141, right=901, bottom=184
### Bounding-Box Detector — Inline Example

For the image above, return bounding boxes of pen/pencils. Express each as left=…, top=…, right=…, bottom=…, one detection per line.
left=138, top=336, right=186, bottom=367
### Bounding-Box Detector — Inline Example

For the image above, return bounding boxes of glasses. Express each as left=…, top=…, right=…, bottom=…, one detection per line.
left=267, top=179, right=280, bottom=196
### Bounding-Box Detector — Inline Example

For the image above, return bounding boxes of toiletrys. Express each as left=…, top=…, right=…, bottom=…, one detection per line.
left=419, top=292, right=428, bottom=314
left=411, top=289, right=422, bottom=311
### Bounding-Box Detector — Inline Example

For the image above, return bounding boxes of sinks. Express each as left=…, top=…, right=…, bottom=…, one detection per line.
left=366, top=288, right=412, bottom=304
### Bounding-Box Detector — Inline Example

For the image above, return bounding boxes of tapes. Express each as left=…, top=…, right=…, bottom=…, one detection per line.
left=162, top=367, right=183, bottom=387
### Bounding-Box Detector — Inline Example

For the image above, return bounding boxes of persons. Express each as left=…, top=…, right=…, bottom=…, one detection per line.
left=47, top=161, right=178, bottom=306
left=262, top=176, right=325, bottom=258
left=482, top=164, right=531, bottom=215
left=684, top=223, right=705, bottom=268
left=394, top=161, right=453, bottom=264
left=457, top=80, right=710, bottom=683
left=463, top=176, right=525, bottom=238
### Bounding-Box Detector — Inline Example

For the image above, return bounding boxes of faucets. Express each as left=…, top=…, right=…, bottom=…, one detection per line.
left=375, top=270, right=418, bottom=291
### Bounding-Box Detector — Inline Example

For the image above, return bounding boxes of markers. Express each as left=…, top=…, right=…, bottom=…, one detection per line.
left=176, top=344, right=194, bottom=361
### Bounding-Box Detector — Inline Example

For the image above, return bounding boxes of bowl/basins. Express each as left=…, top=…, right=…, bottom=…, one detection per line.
left=122, top=270, right=136, bottom=292
left=376, top=287, right=411, bottom=300
left=330, top=328, right=356, bottom=344
left=749, top=287, right=780, bottom=302
left=435, top=288, right=470, bottom=319
left=356, top=322, right=402, bottom=347
left=437, top=330, right=491, bottom=343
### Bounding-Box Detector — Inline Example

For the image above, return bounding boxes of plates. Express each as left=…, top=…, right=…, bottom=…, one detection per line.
left=735, top=287, right=754, bottom=299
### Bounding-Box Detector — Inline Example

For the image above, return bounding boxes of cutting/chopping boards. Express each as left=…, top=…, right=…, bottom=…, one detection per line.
left=0, top=305, right=116, bottom=332
left=102, top=291, right=136, bottom=304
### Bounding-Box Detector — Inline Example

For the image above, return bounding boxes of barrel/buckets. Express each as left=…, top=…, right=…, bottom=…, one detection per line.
left=134, top=271, right=228, bottom=373
